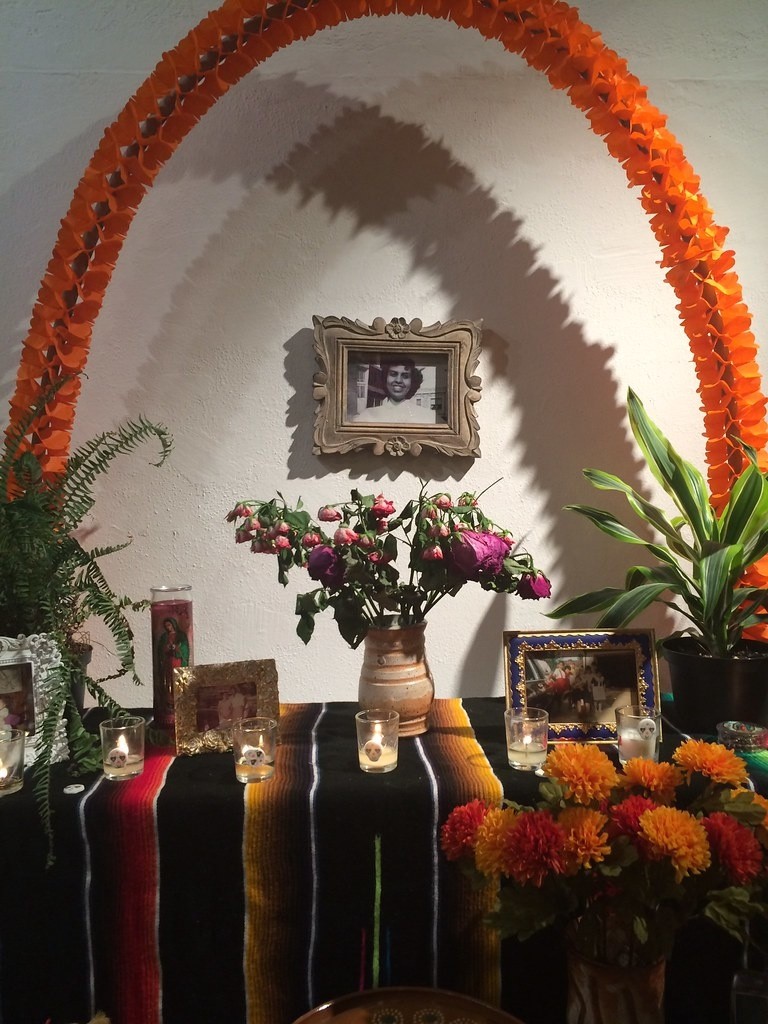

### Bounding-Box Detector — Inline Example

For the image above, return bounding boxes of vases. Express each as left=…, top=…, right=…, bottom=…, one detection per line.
left=560, top=914, right=670, bottom=1024
left=356, top=612, right=436, bottom=741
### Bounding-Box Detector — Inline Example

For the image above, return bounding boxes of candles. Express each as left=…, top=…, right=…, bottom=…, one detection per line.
left=355, top=709, right=401, bottom=775
left=98, top=715, right=147, bottom=783
left=503, top=707, right=550, bottom=773
left=229, top=715, right=281, bottom=782
left=0, top=729, right=23, bottom=798
left=614, top=704, right=662, bottom=769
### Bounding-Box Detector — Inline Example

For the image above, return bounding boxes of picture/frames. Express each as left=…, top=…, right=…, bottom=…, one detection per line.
left=171, top=658, right=285, bottom=760
left=309, top=311, right=488, bottom=460
left=501, top=626, right=666, bottom=746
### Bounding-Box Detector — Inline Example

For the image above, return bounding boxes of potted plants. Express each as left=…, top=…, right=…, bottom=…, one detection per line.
left=2, top=369, right=143, bottom=870
left=540, top=382, right=768, bottom=737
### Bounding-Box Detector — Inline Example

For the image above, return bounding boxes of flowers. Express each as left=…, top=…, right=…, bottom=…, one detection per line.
left=224, top=472, right=553, bottom=654
left=434, top=738, right=768, bottom=967
